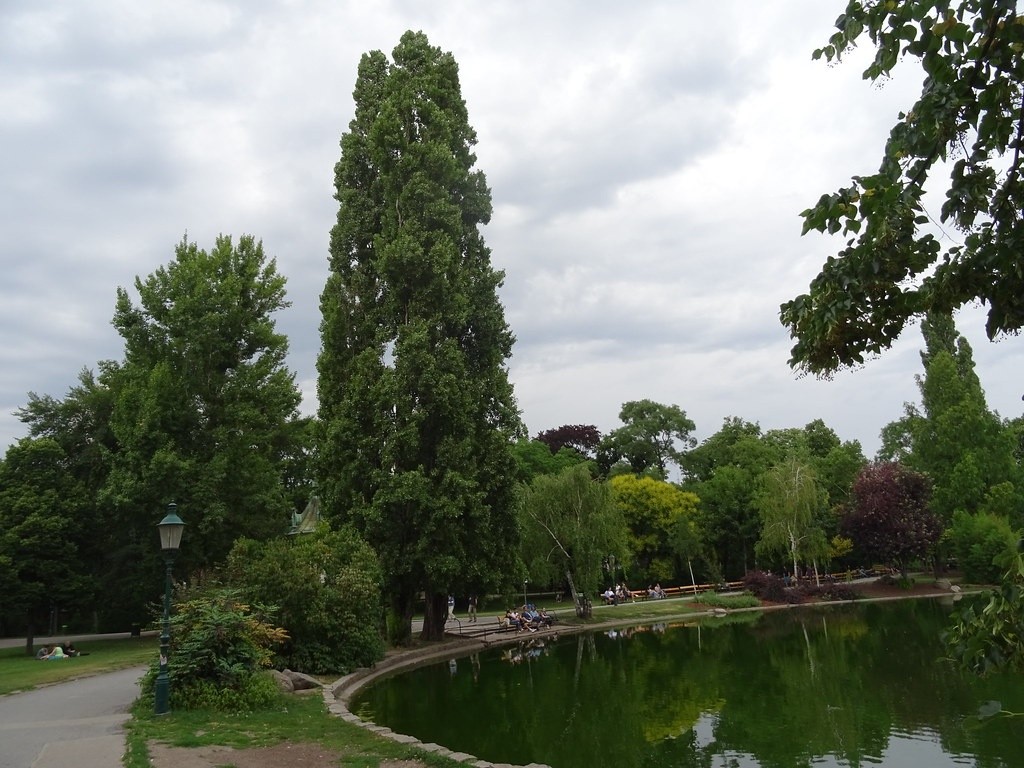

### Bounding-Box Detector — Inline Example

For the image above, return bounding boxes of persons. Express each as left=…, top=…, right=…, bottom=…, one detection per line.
left=646, top=583, right=667, bottom=599
left=555, top=593, right=563, bottom=603
left=760, top=568, right=773, bottom=575
left=797, top=565, right=815, bottom=585
left=35, top=640, right=89, bottom=660
left=783, top=566, right=792, bottom=587
left=504, top=602, right=553, bottom=633
left=609, top=622, right=665, bottom=640
left=448, top=589, right=457, bottom=619
left=467, top=594, right=478, bottom=622
left=846, top=565, right=899, bottom=583
left=604, top=583, right=639, bottom=605
left=501, top=637, right=549, bottom=667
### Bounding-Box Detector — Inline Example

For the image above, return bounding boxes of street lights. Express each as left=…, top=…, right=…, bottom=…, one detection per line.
left=523, top=579, right=529, bottom=606
left=154, top=499, right=187, bottom=714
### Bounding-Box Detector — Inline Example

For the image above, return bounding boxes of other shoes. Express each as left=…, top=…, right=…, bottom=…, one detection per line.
left=531, top=629, right=537, bottom=632
left=521, top=629, right=524, bottom=632
left=469, top=620, right=472, bottom=622
left=518, top=631, right=521, bottom=633
left=474, top=620, right=477, bottom=622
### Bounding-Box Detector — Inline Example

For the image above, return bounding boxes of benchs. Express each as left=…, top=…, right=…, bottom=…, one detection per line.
left=679, top=585, right=703, bottom=597
left=665, top=588, right=681, bottom=598
left=601, top=594, right=607, bottom=605
left=497, top=615, right=545, bottom=636
left=630, top=591, right=648, bottom=601
left=699, top=584, right=721, bottom=594
left=725, top=582, right=746, bottom=591
left=800, top=569, right=875, bottom=585
